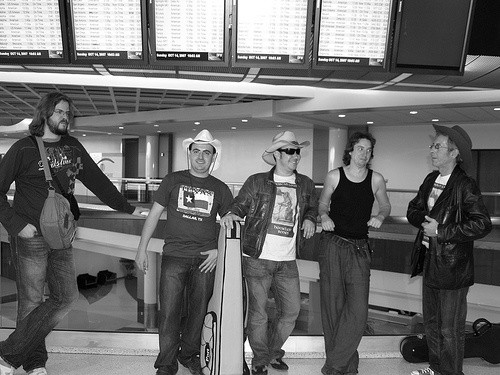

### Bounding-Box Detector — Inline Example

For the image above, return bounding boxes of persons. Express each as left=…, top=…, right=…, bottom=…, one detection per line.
left=406, top=124, right=493, bottom=375
left=135, top=129, right=235, bottom=375
left=0, top=92, right=150, bottom=375
left=220, top=131, right=319, bottom=375
left=319, top=131, right=392, bottom=375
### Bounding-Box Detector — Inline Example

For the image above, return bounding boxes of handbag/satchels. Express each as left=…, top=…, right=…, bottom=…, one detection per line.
left=40, top=190, right=77, bottom=250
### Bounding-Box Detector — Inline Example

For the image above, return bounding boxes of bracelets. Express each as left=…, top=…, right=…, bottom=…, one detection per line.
left=435, top=229, right=438, bottom=234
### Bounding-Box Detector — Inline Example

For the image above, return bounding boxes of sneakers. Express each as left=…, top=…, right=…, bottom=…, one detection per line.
left=410, top=367, right=438, bottom=375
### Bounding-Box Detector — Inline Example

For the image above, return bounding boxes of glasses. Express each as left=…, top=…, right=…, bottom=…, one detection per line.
left=429, top=144, right=452, bottom=152
left=277, top=149, right=300, bottom=155
left=54, top=109, right=72, bottom=117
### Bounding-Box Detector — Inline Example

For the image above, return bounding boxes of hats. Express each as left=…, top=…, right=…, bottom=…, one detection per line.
left=431, top=123, right=473, bottom=162
left=262, top=131, right=310, bottom=166
left=183, top=130, right=222, bottom=171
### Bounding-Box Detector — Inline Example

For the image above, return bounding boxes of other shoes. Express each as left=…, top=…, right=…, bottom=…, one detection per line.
left=178, top=349, right=209, bottom=375
left=0, top=357, right=15, bottom=375
left=252, top=365, right=268, bottom=375
left=321, top=363, right=343, bottom=375
left=154, top=361, right=177, bottom=375
left=270, top=359, right=288, bottom=371
left=28, top=367, right=48, bottom=375
